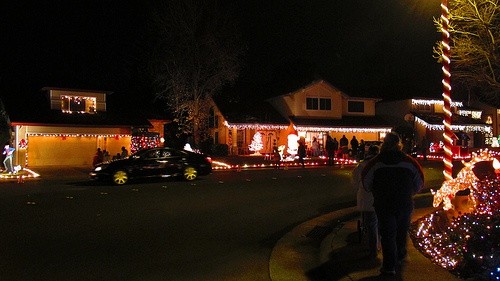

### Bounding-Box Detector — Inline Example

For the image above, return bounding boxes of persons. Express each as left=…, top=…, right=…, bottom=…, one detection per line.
left=351, top=145, right=381, bottom=266
left=93, top=146, right=128, bottom=166
left=274, top=149, right=281, bottom=169
left=313, top=135, right=380, bottom=166
left=298, top=142, right=306, bottom=166
left=2, top=145, right=16, bottom=174
left=361, top=134, right=425, bottom=281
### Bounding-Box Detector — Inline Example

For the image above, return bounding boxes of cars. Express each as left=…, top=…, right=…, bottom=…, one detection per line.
left=87, top=147, right=213, bottom=186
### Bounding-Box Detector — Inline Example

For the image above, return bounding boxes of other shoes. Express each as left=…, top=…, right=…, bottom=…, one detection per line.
left=380, top=269, right=400, bottom=281
left=368, top=259, right=380, bottom=268
